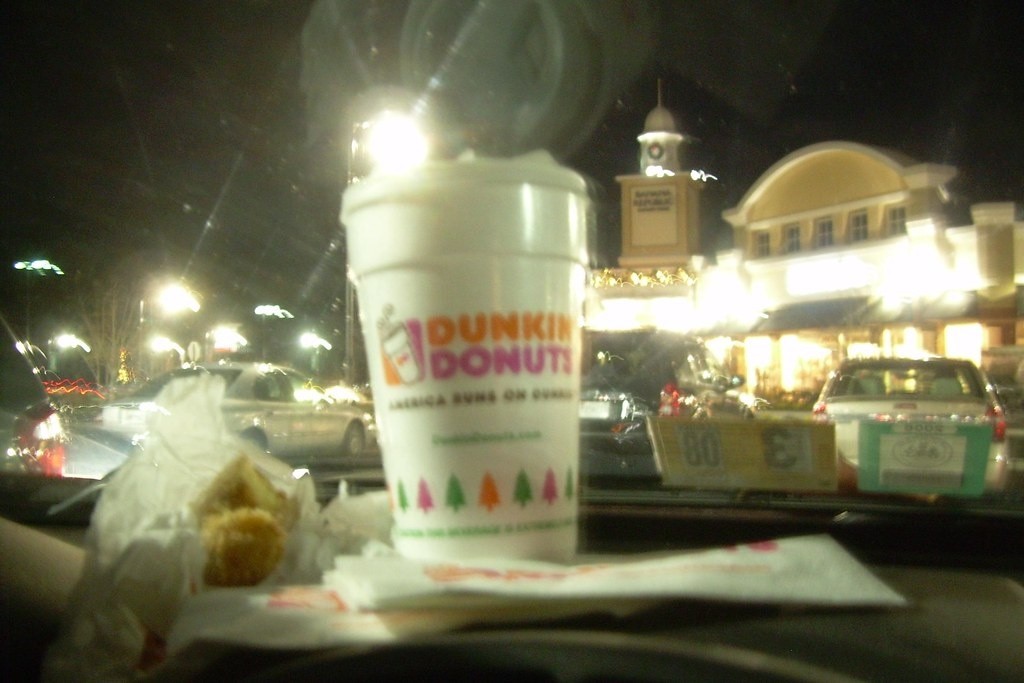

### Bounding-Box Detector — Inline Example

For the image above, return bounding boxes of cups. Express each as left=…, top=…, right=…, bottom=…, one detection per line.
left=342, top=160, right=586, bottom=572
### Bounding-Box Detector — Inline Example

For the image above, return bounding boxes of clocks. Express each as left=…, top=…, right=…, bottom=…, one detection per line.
left=648, top=144, right=664, bottom=160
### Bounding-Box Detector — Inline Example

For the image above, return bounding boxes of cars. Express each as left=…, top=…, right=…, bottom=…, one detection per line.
left=811, top=350, right=1010, bottom=498
left=578, top=328, right=790, bottom=479
left=99, top=360, right=377, bottom=460
left=1, top=310, right=328, bottom=528
